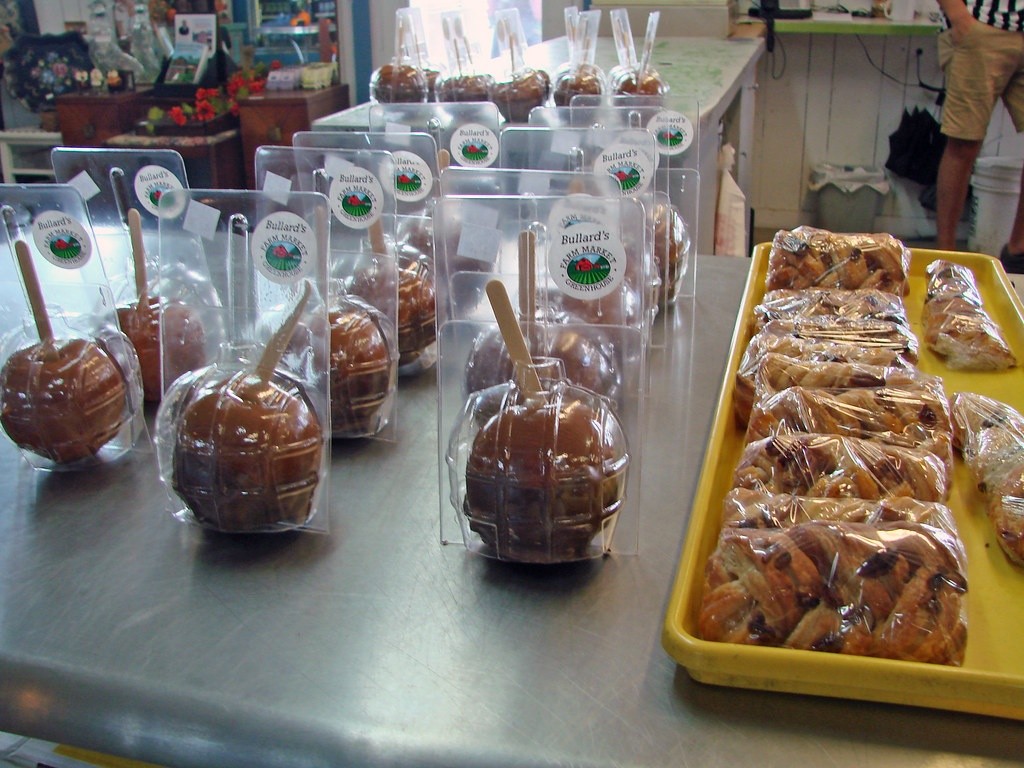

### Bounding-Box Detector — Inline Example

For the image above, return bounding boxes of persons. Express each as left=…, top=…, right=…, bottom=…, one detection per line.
left=934, top=0, right=1023, bottom=273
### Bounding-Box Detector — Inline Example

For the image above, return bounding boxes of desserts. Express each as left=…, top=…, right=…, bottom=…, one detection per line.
left=0, top=64, right=690, bottom=567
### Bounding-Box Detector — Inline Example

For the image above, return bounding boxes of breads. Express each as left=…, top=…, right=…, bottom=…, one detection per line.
left=700, top=220, right=1024, bottom=670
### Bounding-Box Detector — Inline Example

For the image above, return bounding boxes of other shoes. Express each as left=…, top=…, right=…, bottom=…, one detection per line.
left=998, top=242, right=1024, bottom=274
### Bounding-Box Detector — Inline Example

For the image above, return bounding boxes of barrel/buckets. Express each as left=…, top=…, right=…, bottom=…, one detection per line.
left=968, top=157, right=1024, bottom=258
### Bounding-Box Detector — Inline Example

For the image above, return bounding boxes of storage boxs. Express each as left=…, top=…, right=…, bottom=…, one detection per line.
left=268, top=62, right=336, bottom=91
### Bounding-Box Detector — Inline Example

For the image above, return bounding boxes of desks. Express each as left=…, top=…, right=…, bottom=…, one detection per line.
left=0, top=223, right=1024, bottom=768
left=0, top=125, right=63, bottom=184
left=735, top=13, right=943, bottom=38
left=304, top=36, right=766, bottom=255
left=106, top=129, right=244, bottom=234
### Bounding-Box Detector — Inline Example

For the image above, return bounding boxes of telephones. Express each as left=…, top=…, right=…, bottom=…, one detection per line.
left=747, top=0, right=812, bottom=20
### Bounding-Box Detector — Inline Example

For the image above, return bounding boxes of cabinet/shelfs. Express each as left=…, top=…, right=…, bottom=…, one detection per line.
left=57, top=83, right=155, bottom=149
left=237, top=84, right=350, bottom=224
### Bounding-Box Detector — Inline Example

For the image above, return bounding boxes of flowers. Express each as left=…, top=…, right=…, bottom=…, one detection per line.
left=147, top=58, right=283, bottom=135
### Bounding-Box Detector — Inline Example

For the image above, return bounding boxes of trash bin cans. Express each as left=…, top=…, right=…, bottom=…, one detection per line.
left=808, top=159, right=891, bottom=237
